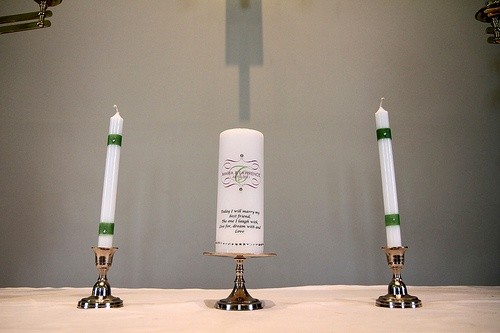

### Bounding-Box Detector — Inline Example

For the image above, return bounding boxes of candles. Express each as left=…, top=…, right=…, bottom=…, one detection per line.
left=98, top=105, right=124, bottom=250
left=374, top=97, right=406, bottom=247
left=214, top=125, right=268, bottom=252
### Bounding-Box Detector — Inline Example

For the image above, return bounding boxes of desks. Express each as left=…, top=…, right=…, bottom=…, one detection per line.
left=0, top=279, right=500, bottom=333
